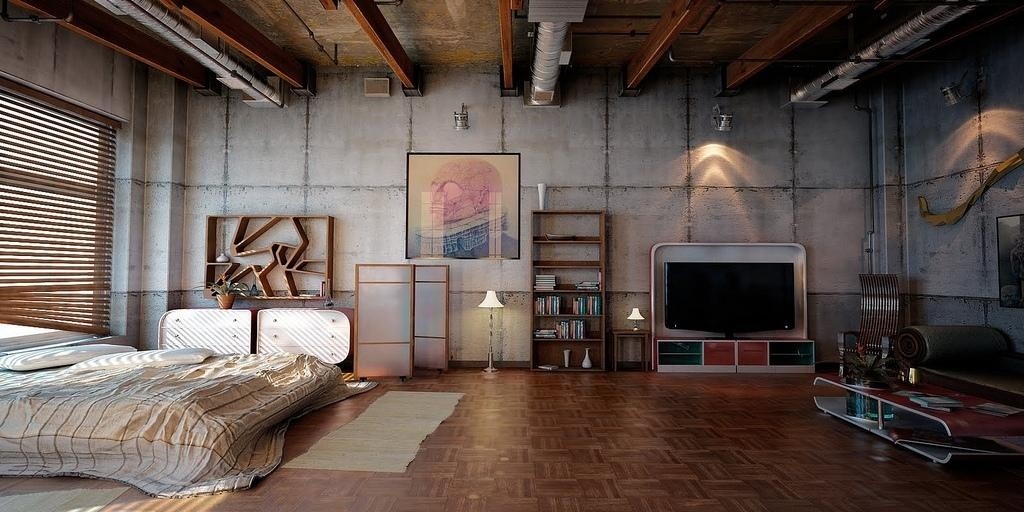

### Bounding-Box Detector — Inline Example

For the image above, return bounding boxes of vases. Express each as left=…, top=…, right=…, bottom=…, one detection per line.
left=582, top=347, right=593, bottom=368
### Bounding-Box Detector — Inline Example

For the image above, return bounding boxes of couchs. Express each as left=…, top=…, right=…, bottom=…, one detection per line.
left=892, top=323, right=1024, bottom=408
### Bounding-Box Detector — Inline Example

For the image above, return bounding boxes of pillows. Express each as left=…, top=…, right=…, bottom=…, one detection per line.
left=2, top=341, right=216, bottom=376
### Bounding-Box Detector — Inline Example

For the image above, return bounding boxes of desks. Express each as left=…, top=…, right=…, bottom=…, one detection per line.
left=613, top=330, right=650, bottom=372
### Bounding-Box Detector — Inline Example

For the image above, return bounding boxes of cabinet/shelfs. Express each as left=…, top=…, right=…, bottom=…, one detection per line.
left=203, top=213, right=333, bottom=300
left=529, top=207, right=608, bottom=373
left=654, top=335, right=816, bottom=374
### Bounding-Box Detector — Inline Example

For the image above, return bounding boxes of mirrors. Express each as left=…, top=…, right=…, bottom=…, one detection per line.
left=996, top=211, right=1024, bottom=309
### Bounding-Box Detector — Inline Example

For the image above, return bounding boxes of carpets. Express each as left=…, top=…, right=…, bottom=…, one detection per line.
left=280, top=390, right=468, bottom=475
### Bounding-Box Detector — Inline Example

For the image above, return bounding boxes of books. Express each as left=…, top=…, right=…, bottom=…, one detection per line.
left=534, top=273, right=602, bottom=339
left=925, top=407, right=953, bottom=414
left=908, top=394, right=963, bottom=408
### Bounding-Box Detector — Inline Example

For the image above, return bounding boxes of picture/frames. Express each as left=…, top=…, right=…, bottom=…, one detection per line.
left=405, top=150, right=521, bottom=260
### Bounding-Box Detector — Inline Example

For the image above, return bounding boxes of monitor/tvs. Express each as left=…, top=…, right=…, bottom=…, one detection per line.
left=663, top=261, right=795, bottom=340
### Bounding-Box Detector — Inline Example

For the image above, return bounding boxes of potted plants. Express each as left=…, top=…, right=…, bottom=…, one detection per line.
left=207, top=274, right=249, bottom=309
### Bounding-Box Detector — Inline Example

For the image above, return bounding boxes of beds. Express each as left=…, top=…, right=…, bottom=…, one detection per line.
left=0, top=345, right=340, bottom=495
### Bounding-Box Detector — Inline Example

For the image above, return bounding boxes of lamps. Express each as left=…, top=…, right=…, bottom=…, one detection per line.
left=453, top=102, right=470, bottom=130
left=477, top=289, right=505, bottom=372
left=627, top=307, right=645, bottom=330
left=713, top=104, right=733, bottom=132
